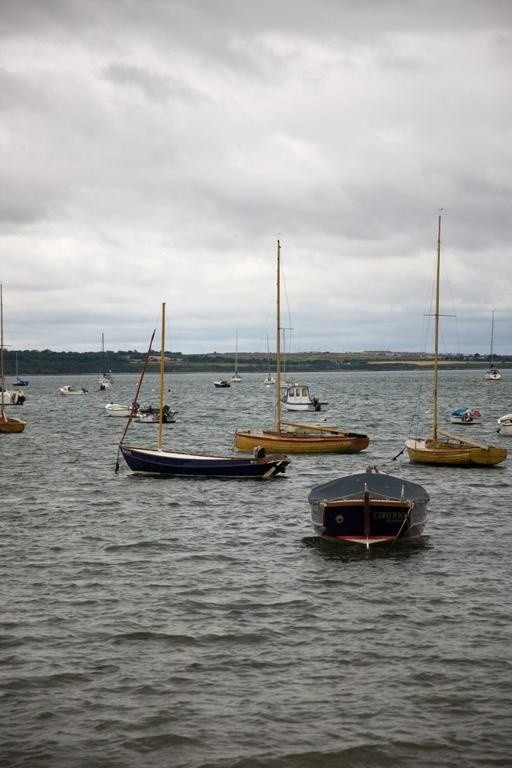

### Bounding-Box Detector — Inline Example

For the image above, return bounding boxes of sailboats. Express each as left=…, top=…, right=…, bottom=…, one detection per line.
left=0, top=282, right=30, bottom=434
left=233, top=239, right=369, bottom=456
left=485, top=310, right=502, bottom=381
left=97, top=332, right=115, bottom=391
left=231, top=328, right=243, bottom=382
left=113, top=302, right=290, bottom=482
left=403, top=213, right=508, bottom=469
left=263, top=332, right=276, bottom=385
left=280, top=329, right=298, bottom=389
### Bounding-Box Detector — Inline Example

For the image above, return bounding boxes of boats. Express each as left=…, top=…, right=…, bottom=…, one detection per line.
left=306, top=466, right=429, bottom=549
left=55, top=386, right=88, bottom=395
left=448, top=406, right=483, bottom=425
left=104, top=402, right=178, bottom=423
left=214, top=379, right=230, bottom=387
left=496, top=413, right=511, bottom=437
left=280, top=386, right=329, bottom=412
